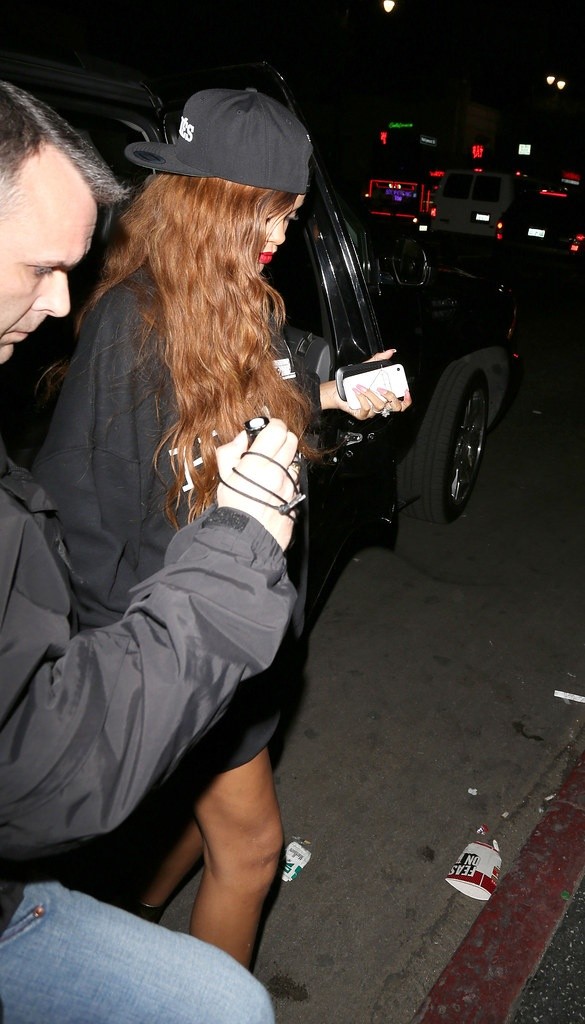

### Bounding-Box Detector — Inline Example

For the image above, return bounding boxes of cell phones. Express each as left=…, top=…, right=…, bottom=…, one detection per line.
left=343, top=364, right=410, bottom=410
left=336, top=360, right=392, bottom=402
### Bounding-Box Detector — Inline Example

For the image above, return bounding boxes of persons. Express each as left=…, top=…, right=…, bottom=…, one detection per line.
left=32, top=86, right=413, bottom=972
left=0, top=79, right=275, bottom=1022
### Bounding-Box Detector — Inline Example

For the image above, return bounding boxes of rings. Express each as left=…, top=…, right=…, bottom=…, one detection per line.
left=371, top=404, right=390, bottom=418
left=289, top=460, right=301, bottom=473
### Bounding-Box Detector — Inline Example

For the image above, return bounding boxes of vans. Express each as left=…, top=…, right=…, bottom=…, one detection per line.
left=428, top=168, right=525, bottom=256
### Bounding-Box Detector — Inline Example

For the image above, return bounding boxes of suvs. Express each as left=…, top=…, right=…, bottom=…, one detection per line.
left=0, top=47, right=520, bottom=647
left=497, top=182, right=585, bottom=273
